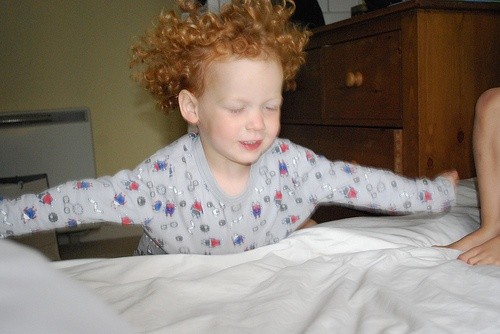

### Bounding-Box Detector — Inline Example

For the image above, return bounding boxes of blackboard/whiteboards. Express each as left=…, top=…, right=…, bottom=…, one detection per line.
left=0, top=107, right=100, bottom=233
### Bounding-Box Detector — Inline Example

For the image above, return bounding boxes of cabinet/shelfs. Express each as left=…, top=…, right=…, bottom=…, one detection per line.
left=279, top=0, right=500, bottom=224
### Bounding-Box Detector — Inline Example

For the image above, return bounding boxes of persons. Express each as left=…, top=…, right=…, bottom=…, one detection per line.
left=431, top=89, right=500, bottom=267
left=0, top=0, right=459, bottom=256
